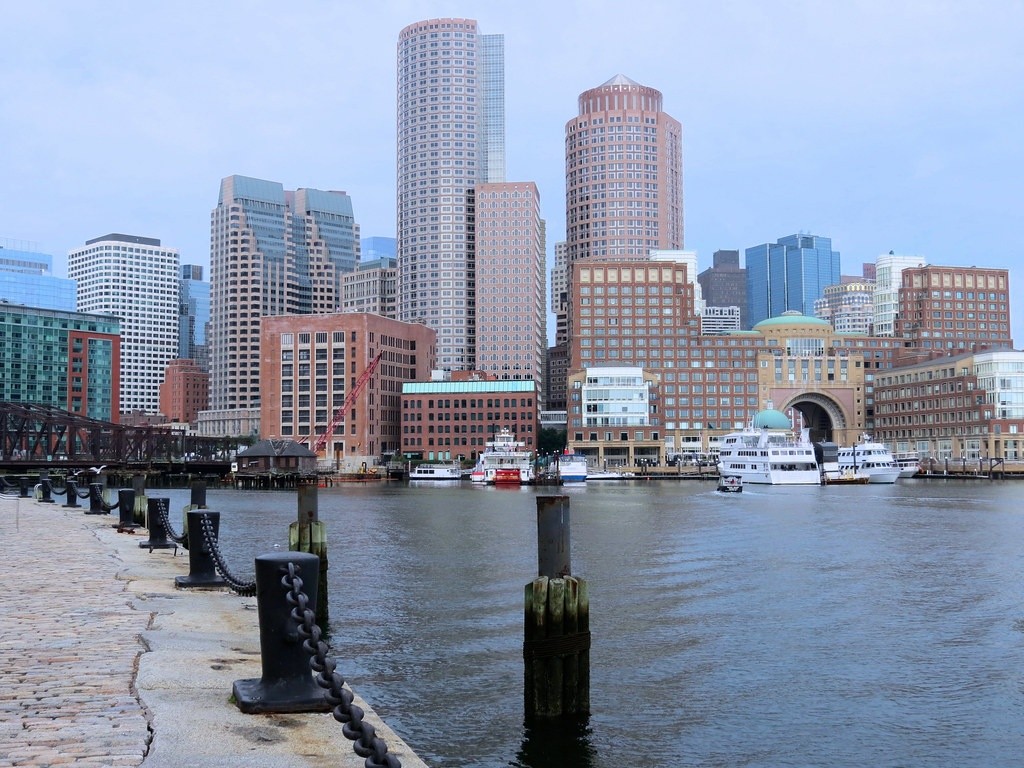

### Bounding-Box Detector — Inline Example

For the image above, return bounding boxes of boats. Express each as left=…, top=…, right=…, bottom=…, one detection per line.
left=716, top=408, right=822, bottom=487
left=538, top=453, right=590, bottom=482
left=836, top=431, right=901, bottom=484
left=470, top=428, right=534, bottom=486
left=386, top=460, right=405, bottom=481
left=410, top=460, right=463, bottom=479
left=891, top=442, right=920, bottom=478
left=717, top=473, right=744, bottom=493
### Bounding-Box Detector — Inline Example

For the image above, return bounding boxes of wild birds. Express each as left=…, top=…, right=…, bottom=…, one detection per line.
left=90, top=466, right=107, bottom=474
left=72, top=470, right=85, bottom=476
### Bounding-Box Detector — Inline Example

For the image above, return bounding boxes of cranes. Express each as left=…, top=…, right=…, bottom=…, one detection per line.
left=296, top=348, right=386, bottom=454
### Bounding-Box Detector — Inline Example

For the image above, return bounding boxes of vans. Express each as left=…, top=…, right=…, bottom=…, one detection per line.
left=674, top=451, right=720, bottom=466
